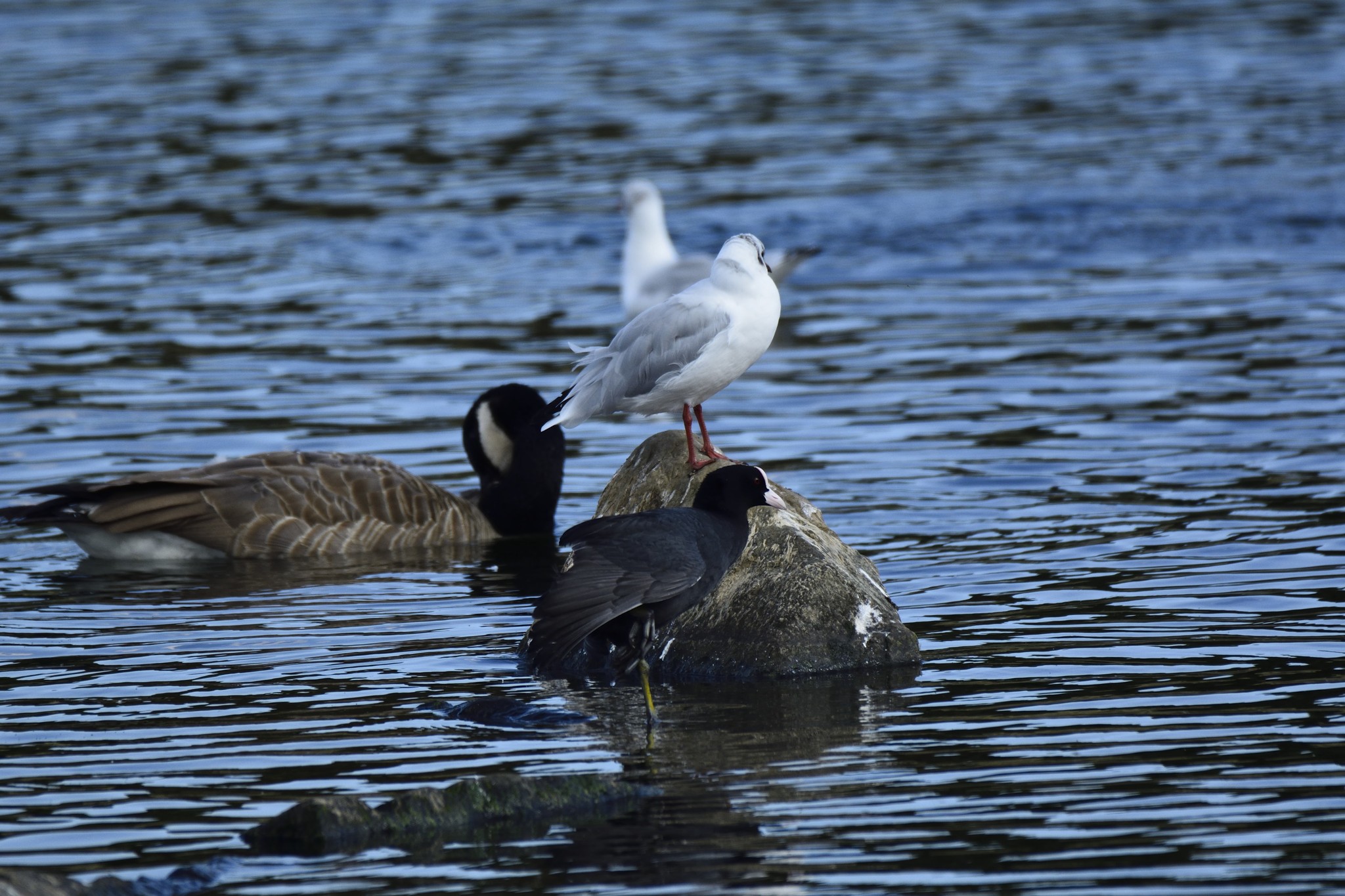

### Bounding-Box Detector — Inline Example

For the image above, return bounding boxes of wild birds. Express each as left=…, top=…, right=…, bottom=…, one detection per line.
left=528, top=464, right=789, bottom=721
left=541, top=178, right=826, bottom=469
left=0, top=384, right=579, bottom=563
